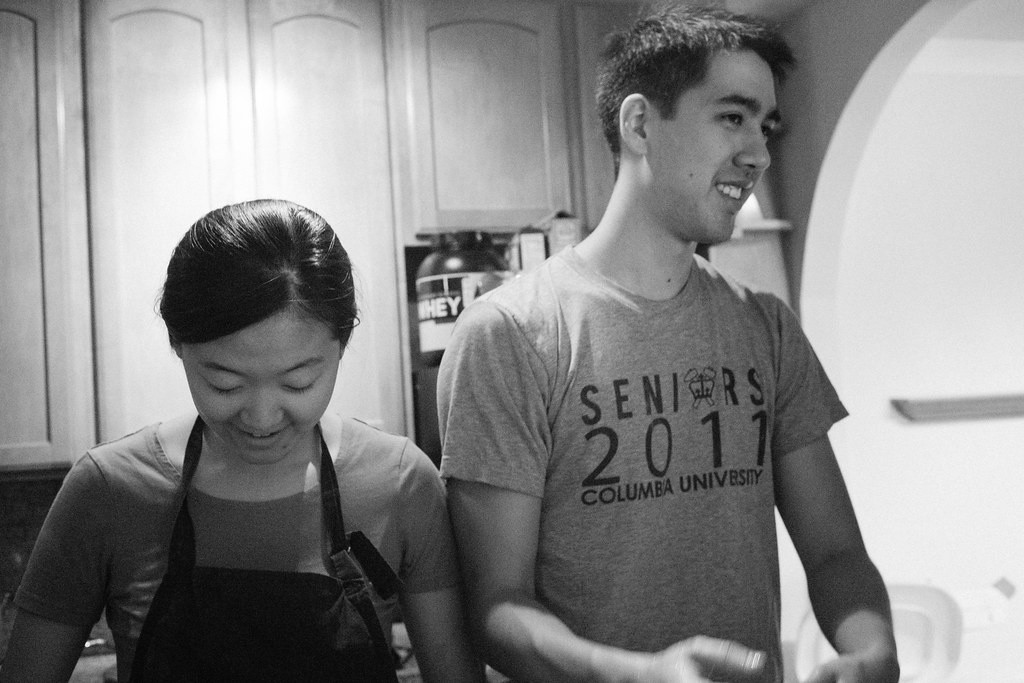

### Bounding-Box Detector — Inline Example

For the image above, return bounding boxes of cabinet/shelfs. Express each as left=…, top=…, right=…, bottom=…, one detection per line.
left=1, top=1, right=795, bottom=472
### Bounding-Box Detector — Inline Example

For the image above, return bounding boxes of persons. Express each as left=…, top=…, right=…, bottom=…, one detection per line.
left=0, top=198, right=484, bottom=683
left=437, top=2, right=900, bottom=683
